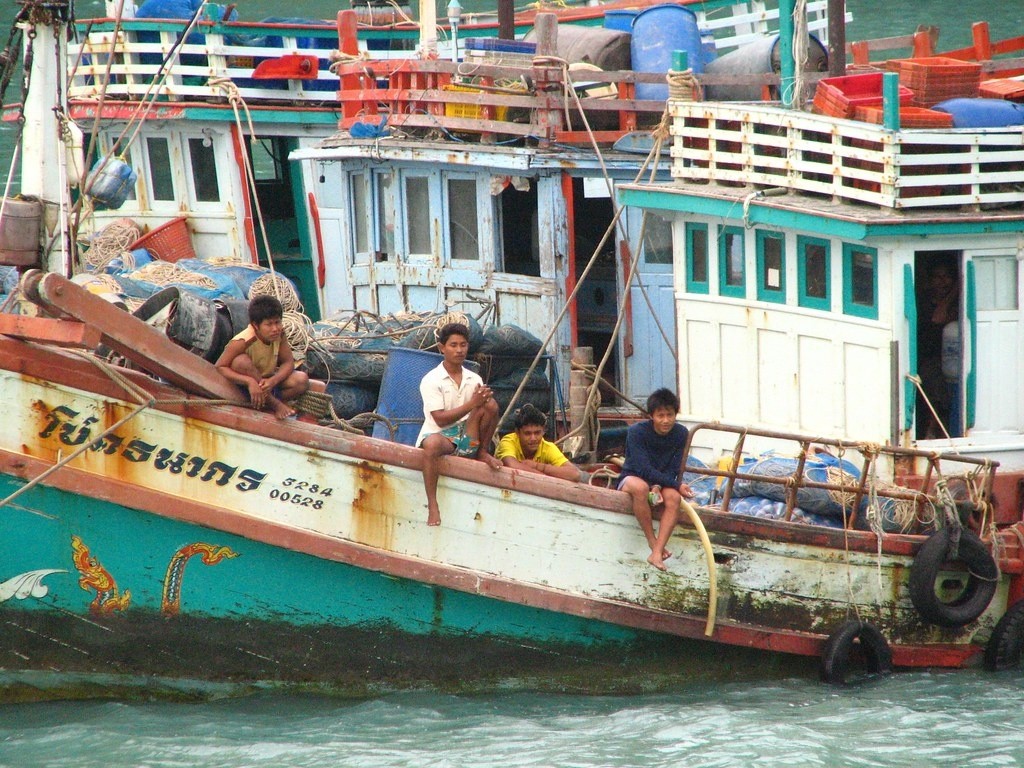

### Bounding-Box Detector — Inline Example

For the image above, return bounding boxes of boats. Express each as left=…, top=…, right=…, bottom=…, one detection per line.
left=1, top=1, right=1023, bottom=689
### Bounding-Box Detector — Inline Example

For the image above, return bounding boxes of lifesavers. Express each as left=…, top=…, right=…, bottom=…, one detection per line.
left=909, top=525, right=997, bottom=627
left=985, top=598, right=1024, bottom=671
left=820, top=621, right=891, bottom=683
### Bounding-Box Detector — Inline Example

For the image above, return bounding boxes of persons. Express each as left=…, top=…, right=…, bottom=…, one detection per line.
left=417, top=324, right=503, bottom=525
left=495, top=409, right=580, bottom=482
left=918, top=258, right=958, bottom=439
left=216, top=295, right=308, bottom=419
left=616, top=388, right=696, bottom=572
